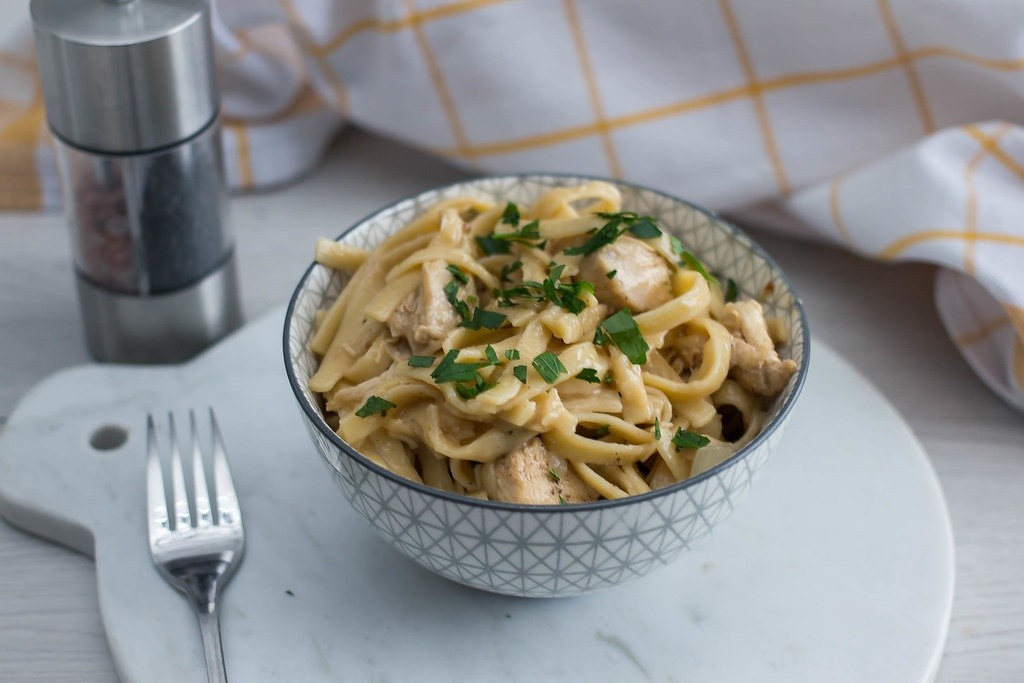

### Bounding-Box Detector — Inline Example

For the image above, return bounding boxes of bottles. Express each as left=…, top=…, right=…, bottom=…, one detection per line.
left=31, top=0, right=246, bottom=368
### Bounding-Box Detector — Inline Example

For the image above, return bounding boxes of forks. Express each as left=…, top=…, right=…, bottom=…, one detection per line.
left=142, top=407, right=244, bottom=683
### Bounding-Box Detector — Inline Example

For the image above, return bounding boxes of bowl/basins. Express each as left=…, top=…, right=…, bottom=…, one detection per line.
left=284, top=175, right=812, bottom=598
